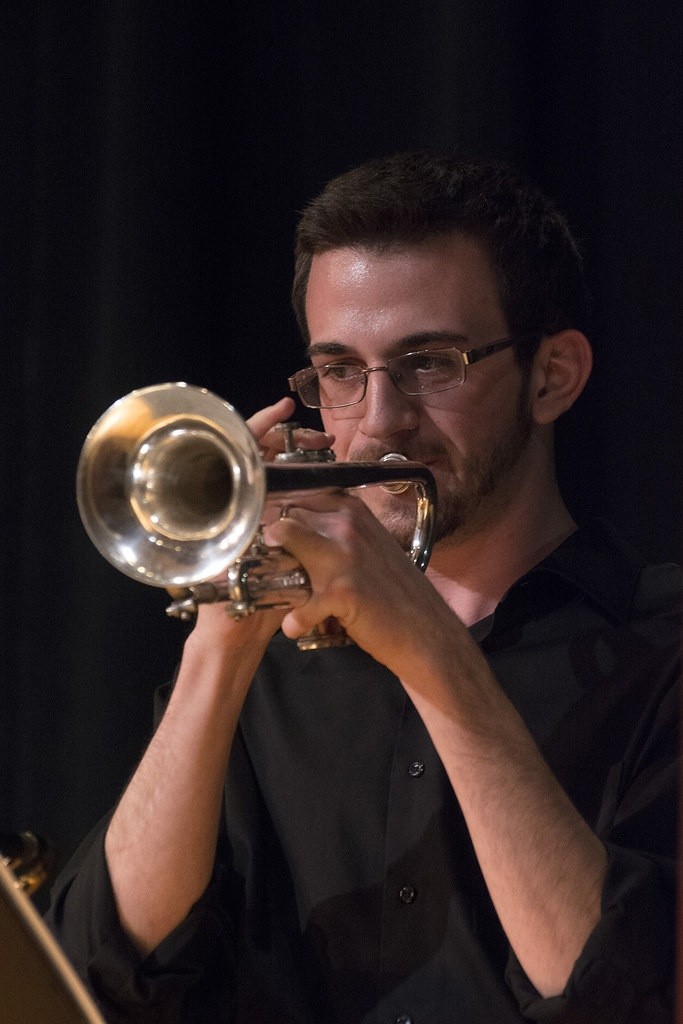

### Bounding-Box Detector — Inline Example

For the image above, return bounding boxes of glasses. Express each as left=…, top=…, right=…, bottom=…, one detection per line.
left=288, top=331, right=532, bottom=410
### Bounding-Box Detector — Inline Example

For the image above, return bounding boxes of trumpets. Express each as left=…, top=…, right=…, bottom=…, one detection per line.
left=75, top=382, right=437, bottom=650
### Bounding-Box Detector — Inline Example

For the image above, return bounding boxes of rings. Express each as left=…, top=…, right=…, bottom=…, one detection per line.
left=280, top=503, right=295, bottom=520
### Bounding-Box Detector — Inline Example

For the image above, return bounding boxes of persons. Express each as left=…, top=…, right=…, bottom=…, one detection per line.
left=38, top=145, right=683, bottom=1024
